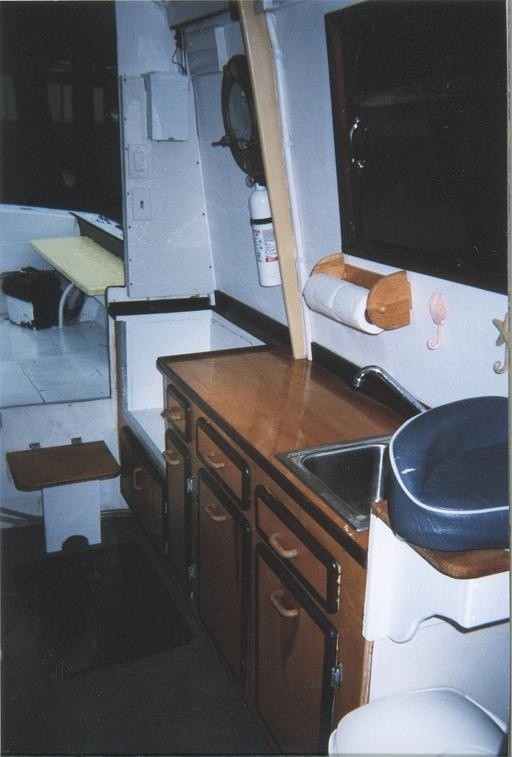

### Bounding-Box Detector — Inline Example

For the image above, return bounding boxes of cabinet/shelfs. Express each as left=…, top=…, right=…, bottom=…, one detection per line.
left=162, top=372, right=365, bottom=757
left=117, top=425, right=169, bottom=567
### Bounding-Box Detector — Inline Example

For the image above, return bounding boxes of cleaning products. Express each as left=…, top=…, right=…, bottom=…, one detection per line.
left=249, top=184, right=281, bottom=289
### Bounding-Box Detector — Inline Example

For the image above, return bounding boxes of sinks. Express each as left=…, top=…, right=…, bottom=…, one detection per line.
left=273, top=435, right=391, bottom=535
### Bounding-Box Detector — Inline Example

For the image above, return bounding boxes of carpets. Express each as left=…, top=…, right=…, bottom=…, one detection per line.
left=12, top=539, right=197, bottom=684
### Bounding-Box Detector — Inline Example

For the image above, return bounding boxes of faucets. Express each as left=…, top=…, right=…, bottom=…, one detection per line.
left=354, top=365, right=426, bottom=414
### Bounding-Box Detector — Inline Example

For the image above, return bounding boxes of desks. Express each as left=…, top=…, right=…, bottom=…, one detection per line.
left=29, top=235, right=127, bottom=330
